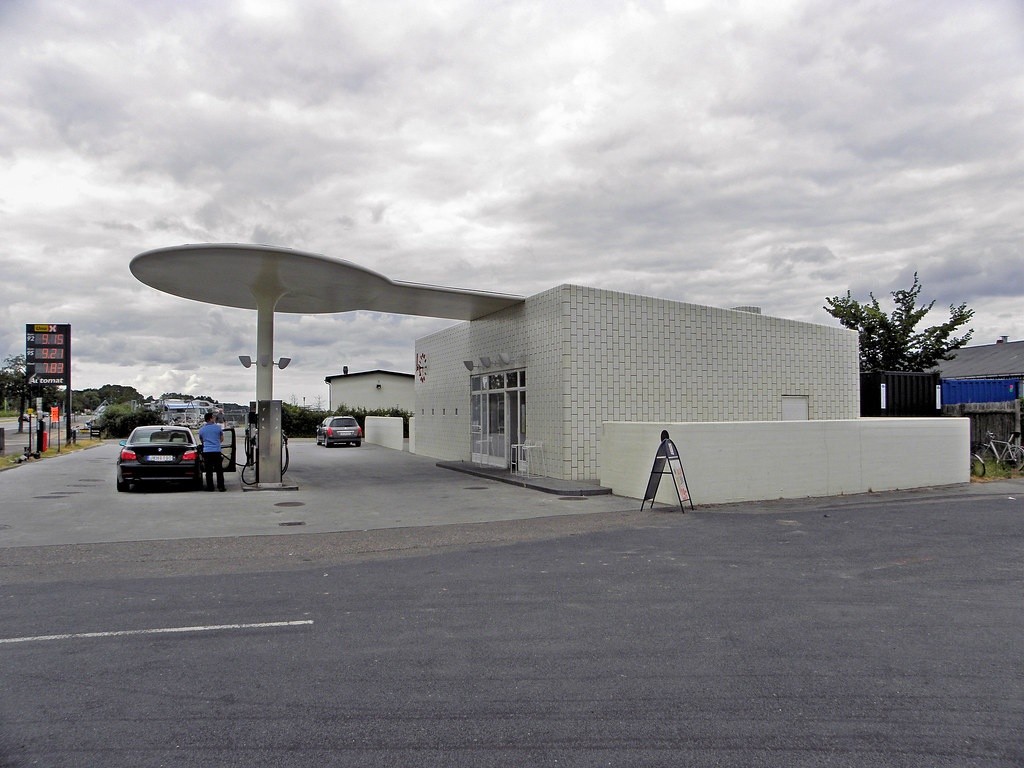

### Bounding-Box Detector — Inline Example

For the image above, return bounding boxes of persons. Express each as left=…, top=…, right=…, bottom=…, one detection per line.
left=216, top=409, right=227, bottom=429
left=199, top=413, right=227, bottom=492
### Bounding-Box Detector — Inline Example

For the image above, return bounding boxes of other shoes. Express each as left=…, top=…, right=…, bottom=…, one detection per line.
left=206, top=487, right=214, bottom=491
left=219, top=488, right=226, bottom=492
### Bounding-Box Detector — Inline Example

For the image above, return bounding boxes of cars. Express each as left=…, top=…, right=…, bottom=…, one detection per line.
left=18, top=412, right=50, bottom=422
left=117, top=425, right=237, bottom=492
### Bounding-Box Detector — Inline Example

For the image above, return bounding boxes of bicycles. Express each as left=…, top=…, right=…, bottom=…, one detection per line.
left=974, top=430, right=1024, bottom=473
left=970, top=440, right=986, bottom=477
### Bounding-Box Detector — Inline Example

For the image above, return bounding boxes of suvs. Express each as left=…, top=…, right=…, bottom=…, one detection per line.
left=316, top=414, right=362, bottom=448
